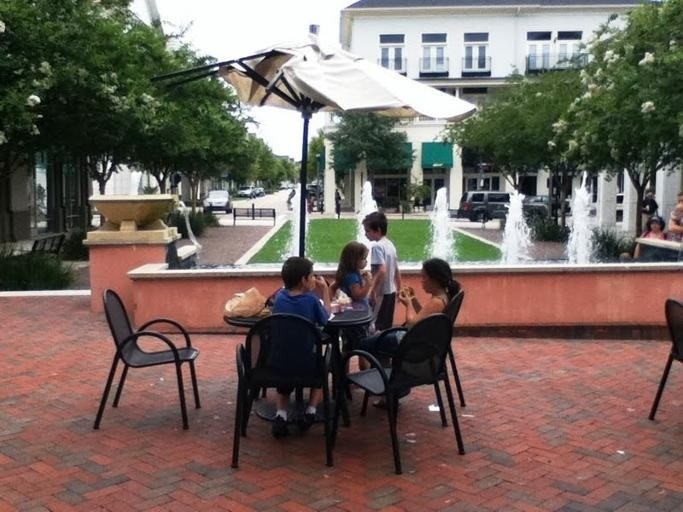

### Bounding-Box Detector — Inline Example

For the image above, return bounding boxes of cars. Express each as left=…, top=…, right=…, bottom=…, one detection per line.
left=615, top=193, right=625, bottom=217
left=287, top=185, right=322, bottom=212
left=525, top=195, right=570, bottom=218
left=278, top=182, right=301, bottom=189
left=255, top=187, right=265, bottom=196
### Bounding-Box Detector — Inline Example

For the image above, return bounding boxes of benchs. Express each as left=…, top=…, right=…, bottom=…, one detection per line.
left=232, top=204, right=276, bottom=227
left=9, top=234, right=65, bottom=262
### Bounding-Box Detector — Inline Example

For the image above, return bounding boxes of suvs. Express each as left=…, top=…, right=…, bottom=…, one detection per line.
left=202, top=189, right=233, bottom=214
left=237, top=186, right=256, bottom=200
left=457, top=190, right=549, bottom=224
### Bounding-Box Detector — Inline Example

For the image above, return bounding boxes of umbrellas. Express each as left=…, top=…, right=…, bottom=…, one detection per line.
left=149, top=24, right=481, bottom=257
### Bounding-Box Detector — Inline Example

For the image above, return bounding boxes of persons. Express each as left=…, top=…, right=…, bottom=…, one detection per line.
left=668, top=193, right=683, bottom=242
left=634, top=216, right=672, bottom=259
left=360, top=257, right=462, bottom=407
left=272, top=257, right=331, bottom=442
left=328, top=241, right=373, bottom=371
left=640, top=189, right=658, bottom=231
left=361, top=212, right=402, bottom=328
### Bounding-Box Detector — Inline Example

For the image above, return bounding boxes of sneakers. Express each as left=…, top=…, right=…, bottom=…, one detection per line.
left=271, top=412, right=317, bottom=440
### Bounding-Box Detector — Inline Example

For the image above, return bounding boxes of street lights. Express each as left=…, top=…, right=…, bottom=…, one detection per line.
left=315, top=152, right=321, bottom=212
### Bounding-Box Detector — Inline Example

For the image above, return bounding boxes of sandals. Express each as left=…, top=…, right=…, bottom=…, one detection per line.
left=372, top=397, right=386, bottom=408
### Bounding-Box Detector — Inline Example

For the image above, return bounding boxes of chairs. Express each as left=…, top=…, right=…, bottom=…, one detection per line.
left=230, top=313, right=348, bottom=466
left=648, top=299, right=683, bottom=420
left=327, top=313, right=465, bottom=476
left=93, top=289, right=200, bottom=430
left=360, top=288, right=465, bottom=427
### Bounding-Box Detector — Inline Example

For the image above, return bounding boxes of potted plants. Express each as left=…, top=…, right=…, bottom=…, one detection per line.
left=399, top=176, right=432, bottom=214
left=589, top=223, right=634, bottom=264
left=524, top=208, right=571, bottom=261
